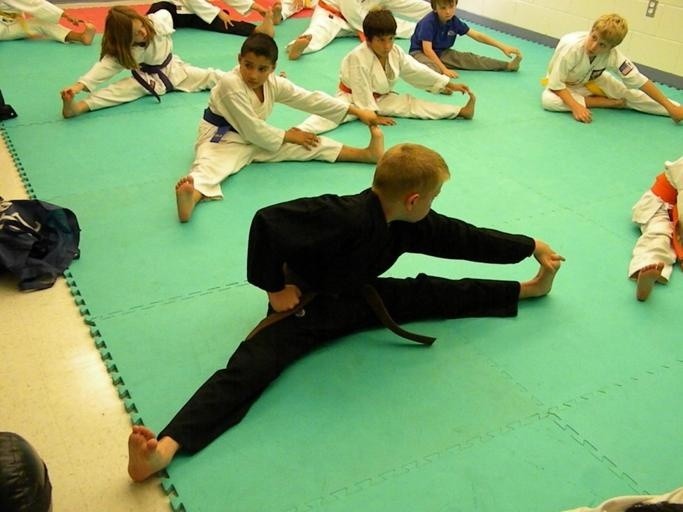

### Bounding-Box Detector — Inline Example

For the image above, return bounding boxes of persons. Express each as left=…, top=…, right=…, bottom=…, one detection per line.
left=146, top=0, right=274, bottom=39
left=0, top=0, right=97, bottom=46
left=271, top=0, right=317, bottom=25
left=541, top=13, right=682, bottom=123
left=562, top=488, right=683, bottom=512
left=174, top=32, right=400, bottom=222
left=285, top=0, right=434, bottom=60
left=60, top=4, right=286, bottom=118
left=129, top=142, right=565, bottom=482
left=288, top=9, right=476, bottom=135
left=629, top=156, right=683, bottom=301
left=409, top=0, right=522, bottom=96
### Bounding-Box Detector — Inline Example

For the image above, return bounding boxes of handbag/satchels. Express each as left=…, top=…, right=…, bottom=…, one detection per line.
left=0, top=198, right=80, bottom=290
